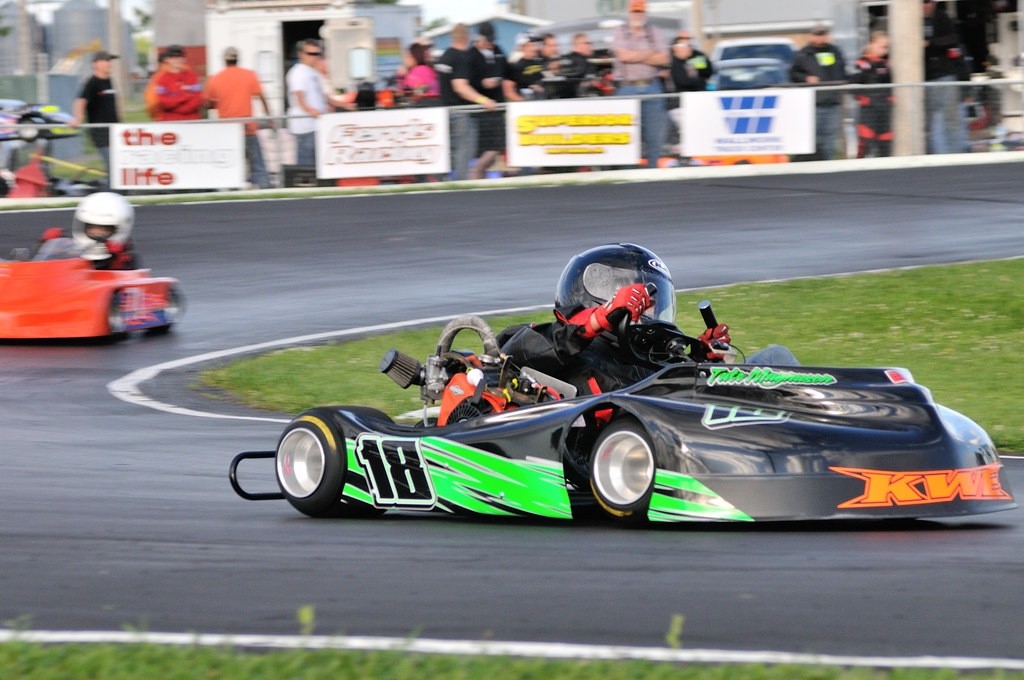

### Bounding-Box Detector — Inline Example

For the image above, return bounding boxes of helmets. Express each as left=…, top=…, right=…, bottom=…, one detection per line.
left=554, top=243, right=677, bottom=325
left=71, top=191, right=134, bottom=250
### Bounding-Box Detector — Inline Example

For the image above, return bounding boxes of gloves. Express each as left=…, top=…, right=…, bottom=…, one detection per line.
left=103, top=240, right=127, bottom=256
left=596, top=283, right=656, bottom=331
left=697, top=324, right=731, bottom=360
left=41, top=227, right=63, bottom=242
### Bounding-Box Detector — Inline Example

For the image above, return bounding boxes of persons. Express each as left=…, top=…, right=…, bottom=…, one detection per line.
left=790, top=25, right=844, bottom=162
left=285, top=39, right=357, bottom=184
left=923, top=0, right=988, bottom=154
left=143, top=44, right=206, bottom=120
left=495, top=241, right=796, bottom=400
left=851, top=30, right=894, bottom=158
left=201, top=48, right=278, bottom=187
left=670, top=32, right=714, bottom=94
left=392, top=19, right=618, bottom=182
left=66, top=50, right=130, bottom=197
left=33, top=191, right=142, bottom=271
left=609, top=0, right=674, bottom=170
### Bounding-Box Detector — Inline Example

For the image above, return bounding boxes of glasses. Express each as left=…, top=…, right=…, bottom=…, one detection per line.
left=302, top=53, right=322, bottom=56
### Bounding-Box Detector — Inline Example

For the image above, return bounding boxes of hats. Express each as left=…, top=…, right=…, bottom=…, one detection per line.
left=409, top=37, right=433, bottom=47
left=629, top=1, right=645, bottom=13
left=224, top=48, right=237, bottom=61
left=166, top=45, right=185, bottom=57
left=813, top=25, right=827, bottom=34
left=91, top=50, right=119, bottom=61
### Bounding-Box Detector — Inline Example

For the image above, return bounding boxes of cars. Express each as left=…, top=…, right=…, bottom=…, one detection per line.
left=707, top=37, right=799, bottom=92
